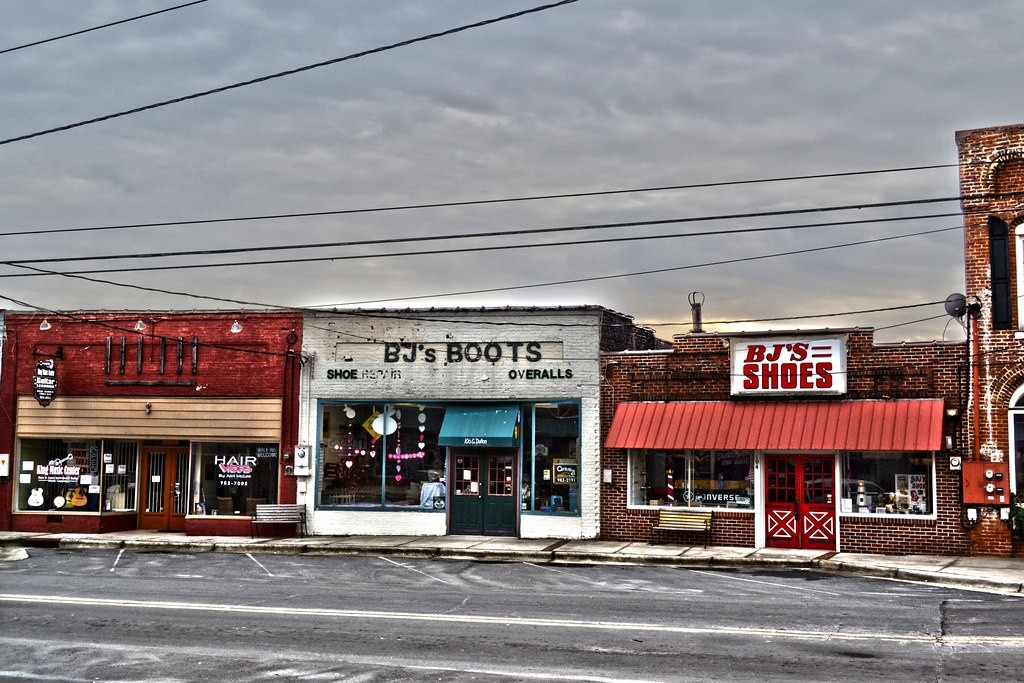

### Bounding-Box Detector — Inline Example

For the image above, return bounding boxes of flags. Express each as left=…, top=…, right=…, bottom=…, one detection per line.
left=749, top=453, right=754, bottom=485
left=666, top=469, right=674, bottom=503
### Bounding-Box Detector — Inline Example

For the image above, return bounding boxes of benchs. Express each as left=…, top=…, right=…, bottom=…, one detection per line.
left=250, top=504, right=309, bottom=540
left=646, top=510, right=715, bottom=550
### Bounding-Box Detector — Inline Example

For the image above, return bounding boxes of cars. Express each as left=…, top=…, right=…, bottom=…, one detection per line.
left=344, top=466, right=444, bottom=508
left=807, top=477, right=894, bottom=515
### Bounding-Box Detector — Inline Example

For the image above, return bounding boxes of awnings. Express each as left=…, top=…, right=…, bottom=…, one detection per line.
left=605, top=396, right=946, bottom=452
left=437, top=405, right=520, bottom=448
